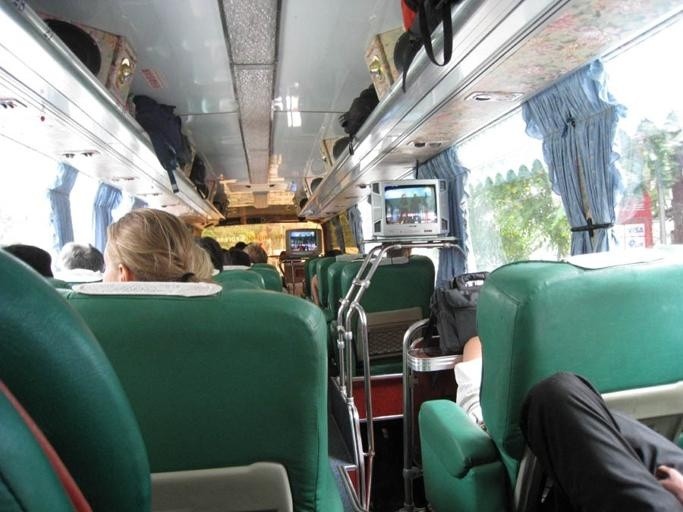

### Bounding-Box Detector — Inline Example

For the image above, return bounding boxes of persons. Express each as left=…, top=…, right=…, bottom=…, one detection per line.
left=397, top=193, right=408, bottom=218
left=518, top=370, right=682, bottom=511
left=410, top=193, right=421, bottom=223
left=451, top=334, right=489, bottom=435
left=421, top=192, right=430, bottom=220
left=99, top=208, right=201, bottom=283
left=1, top=222, right=268, bottom=277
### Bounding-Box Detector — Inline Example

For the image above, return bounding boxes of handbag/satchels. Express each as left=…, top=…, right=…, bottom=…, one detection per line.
left=423, top=272, right=487, bottom=356
left=134, top=95, right=209, bottom=201
left=339, top=83, right=379, bottom=135
left=393, top=1, right=457, bottom=72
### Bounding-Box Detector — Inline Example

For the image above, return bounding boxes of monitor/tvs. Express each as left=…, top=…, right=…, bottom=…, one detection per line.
left=285, top=229, right=322, bottom=256
left=371, top=179, right=450, bottom=236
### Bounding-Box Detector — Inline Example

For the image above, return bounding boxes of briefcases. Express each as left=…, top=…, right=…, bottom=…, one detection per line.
left=36, top=11, right=137, bottom=106
left=365, top=27, right=403, bottom=101
left=320, top=136, right=345, bottom=169
